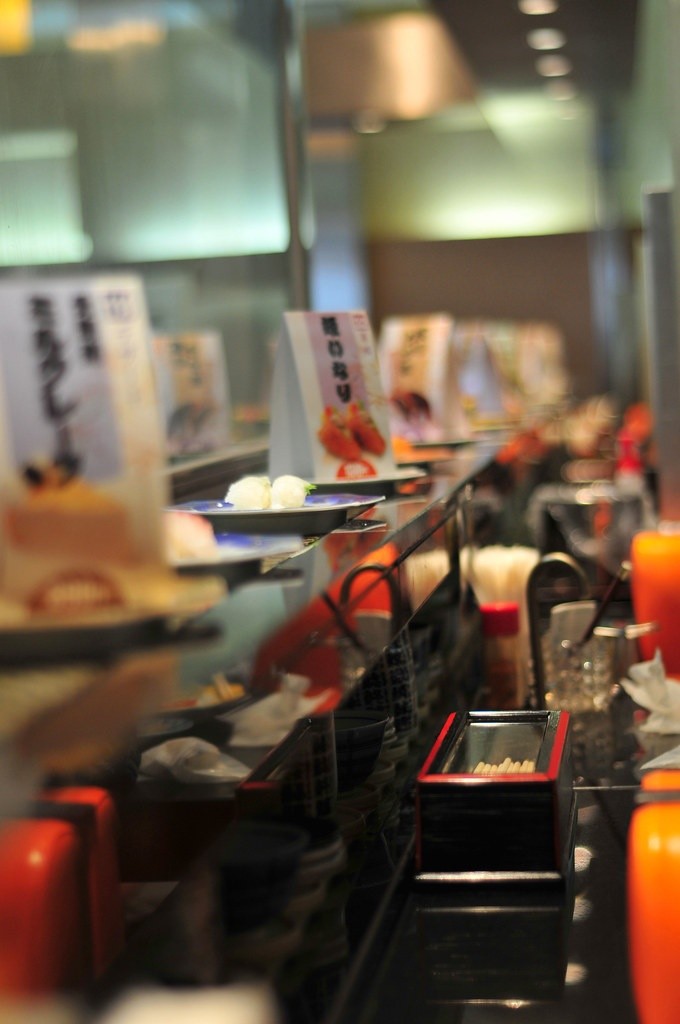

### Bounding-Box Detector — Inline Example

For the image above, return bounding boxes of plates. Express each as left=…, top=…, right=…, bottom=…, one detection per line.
left=397, top=457, right=453, bottom=472
left=181, top=495, right=388, bottom=534
left=172, top=535, right=301, bottom=586
left=312, top=470, right=426, bottom=495
left=412, top=440, right=476, bottom=452
left=139, top=681, right=248, bottom=722
left=0, top=578, right=237, bottom=668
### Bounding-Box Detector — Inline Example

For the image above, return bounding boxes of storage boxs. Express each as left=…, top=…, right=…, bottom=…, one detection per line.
left=416, top=709, right=579, bottom=911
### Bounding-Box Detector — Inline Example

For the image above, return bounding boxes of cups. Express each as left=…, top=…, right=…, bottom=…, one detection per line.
left=541, top=632, right=621, bottom=731
left=629, top=521, right=679, bottom=681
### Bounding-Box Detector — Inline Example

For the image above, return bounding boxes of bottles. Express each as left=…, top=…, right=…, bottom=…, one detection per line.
left=628, top=773, right=680, bottom=1024
